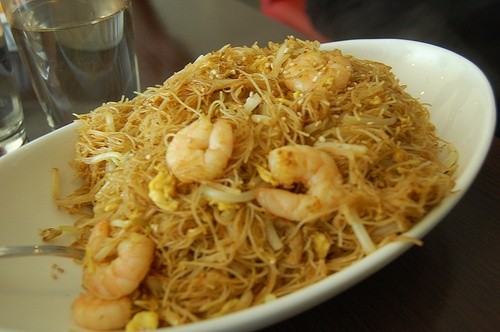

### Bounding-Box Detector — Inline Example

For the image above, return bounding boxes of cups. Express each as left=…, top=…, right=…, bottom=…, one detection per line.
left=0, top=18, right=27, bottom=157
left=0, top=0, right=141, bottom=131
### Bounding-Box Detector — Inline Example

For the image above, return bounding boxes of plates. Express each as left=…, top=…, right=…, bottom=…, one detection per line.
left=0, top=39, right=497, bottom=332
